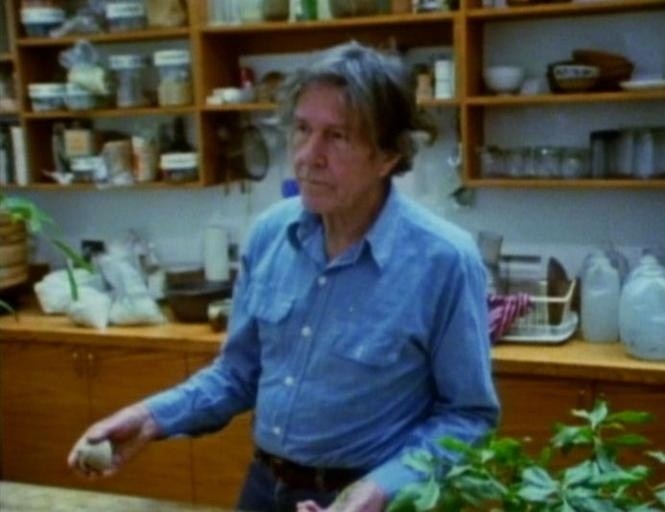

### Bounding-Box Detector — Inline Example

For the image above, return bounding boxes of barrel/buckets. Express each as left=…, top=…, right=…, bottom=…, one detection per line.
left=579, top=246, right=665, bottom=361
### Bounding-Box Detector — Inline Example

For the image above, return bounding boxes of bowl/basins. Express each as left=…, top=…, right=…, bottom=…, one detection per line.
left=551, top=63, right=600, bottom=92
left=479, top=65, right=525, bottom=96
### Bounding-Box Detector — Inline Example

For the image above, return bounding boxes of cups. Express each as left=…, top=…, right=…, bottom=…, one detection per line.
left=206, top=0, right=441, bottom=27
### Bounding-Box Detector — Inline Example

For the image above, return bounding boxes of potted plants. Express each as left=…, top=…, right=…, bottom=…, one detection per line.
left=0, top=196, right=96, bottom=325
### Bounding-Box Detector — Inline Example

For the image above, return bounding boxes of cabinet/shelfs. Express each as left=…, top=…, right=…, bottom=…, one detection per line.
left=1, top=333, right=664, bottom=511
left=0, top=0, right=665, bottom=190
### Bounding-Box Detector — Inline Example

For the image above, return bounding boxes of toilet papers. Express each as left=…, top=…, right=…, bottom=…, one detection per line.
left=9, top=125, right=29, bottom=187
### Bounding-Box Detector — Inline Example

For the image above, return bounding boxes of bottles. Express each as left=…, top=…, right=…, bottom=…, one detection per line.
left=0, top=0, right=201, bottom=187
left=434, top=53, right=454, bottom=99
left=415, top=61, right=434, bottom=102
left=479, top=124, right=664, bottom=181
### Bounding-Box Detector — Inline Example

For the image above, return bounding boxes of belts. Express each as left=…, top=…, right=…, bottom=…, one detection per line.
left=253, top=448, right=365, bottom=491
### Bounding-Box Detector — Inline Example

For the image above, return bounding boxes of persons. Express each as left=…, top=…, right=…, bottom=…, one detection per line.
left=66, top=39, right=501, bottom=512
